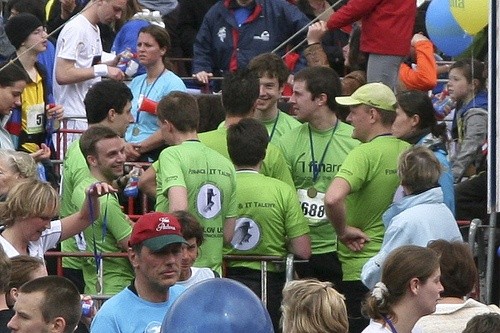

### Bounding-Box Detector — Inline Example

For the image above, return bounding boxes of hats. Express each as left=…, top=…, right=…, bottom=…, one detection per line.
left=128, top=211, right=190, bottom=252
left=5, top=13, right=43, bottom=50
left=334, top=82, right=398, bottom=112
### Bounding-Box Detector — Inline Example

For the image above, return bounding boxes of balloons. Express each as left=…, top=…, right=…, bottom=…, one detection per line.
left=425, top=0, right=473, bottom=57
left=449, top=0, right=489, bottom=35
left=160, top=278, right=274, bottom=333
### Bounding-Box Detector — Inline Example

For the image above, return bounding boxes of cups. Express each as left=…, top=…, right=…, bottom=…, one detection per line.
left=138, top=95, right=157, bottom=114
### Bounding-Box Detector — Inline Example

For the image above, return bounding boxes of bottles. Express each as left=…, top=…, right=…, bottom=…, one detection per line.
left=431, top=90, right=447, bottom=107
left=117, top=48, right=132, bottom=73
left=46, top=104, right=57, bottom=132
left=123, top=164, right=143, bottom=198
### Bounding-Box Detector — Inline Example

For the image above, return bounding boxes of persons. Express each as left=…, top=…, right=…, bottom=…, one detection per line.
left=192, top=0, right=500, bottom=284
left=0, top=0, right=239, bottom=333
left=224, top=82, right=500, bottom=333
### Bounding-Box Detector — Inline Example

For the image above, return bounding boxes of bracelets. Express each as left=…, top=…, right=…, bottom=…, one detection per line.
left=94, top=64, right=108, bottom=78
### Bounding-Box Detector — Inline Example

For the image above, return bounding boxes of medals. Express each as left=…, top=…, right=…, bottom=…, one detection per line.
left=132, top=128, right=139, bottom=136
left=308, top=187, right=317, bottom=198
left=96, top=280, right=102, bottom=292
